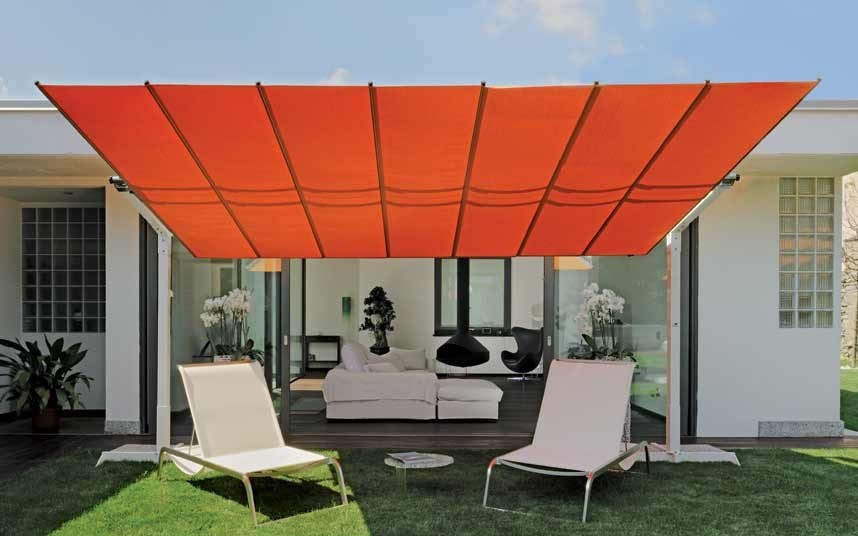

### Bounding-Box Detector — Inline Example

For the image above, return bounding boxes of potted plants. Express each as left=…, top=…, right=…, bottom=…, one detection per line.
left=362, top=283, right=399, bottom=355
left=0, top=336, right=94, bottom=434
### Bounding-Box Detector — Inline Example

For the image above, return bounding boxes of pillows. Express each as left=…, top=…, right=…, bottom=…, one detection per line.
left=342, top=344, right=406, bottom=373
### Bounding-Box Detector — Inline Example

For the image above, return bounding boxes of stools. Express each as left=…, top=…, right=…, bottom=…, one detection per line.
left=436, top=377, right=503, bottom=420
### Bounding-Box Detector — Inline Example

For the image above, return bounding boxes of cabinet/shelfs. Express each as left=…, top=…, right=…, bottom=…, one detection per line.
left=299, top=333, right=341, bottom=378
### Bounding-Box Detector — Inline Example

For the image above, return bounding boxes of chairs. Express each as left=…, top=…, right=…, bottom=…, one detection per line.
left=502, top=326, right=545, bottom=393
left=156, top=362, right=350, bottom=526
left=482, top=359, right=651, bottom=525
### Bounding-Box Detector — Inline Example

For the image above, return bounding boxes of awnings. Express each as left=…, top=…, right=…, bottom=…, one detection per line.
left=35, top=78, right=821, bottom=258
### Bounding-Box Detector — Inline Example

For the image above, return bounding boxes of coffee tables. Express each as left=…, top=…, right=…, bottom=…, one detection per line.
left=384, top=451, right=454, bottom=493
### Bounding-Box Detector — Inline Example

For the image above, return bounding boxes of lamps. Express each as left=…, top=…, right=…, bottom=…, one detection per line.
left=342, top=296, right=353, bottom=315
left=246, top=258, right=282, bottom=272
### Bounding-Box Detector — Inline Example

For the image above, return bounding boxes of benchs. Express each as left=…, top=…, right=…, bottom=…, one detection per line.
left=322, top=342, right=437, bottom=421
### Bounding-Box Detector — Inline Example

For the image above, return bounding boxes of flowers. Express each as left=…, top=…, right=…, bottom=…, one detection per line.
left=197, top=286, right=264, bottom=366
left=568, top=282, right=641, bottom=363
